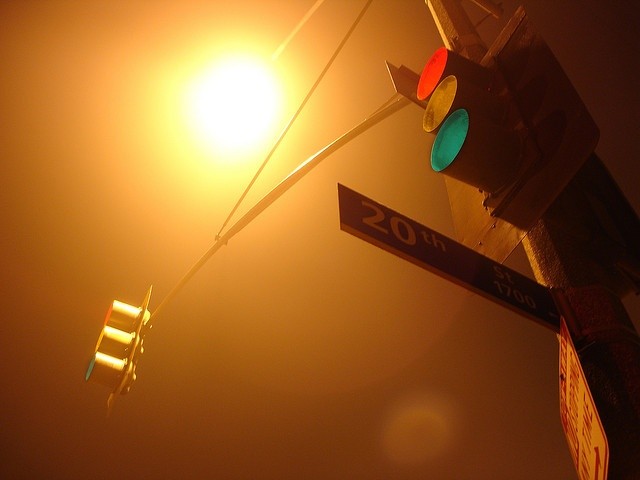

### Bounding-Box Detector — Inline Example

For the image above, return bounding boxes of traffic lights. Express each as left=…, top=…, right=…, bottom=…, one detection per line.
left=417, top=8, right=600, bottom=264
left=82, top=285, right=152, bottom=420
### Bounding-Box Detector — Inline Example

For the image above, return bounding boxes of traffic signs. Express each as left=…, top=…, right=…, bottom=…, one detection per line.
left=337, top=182, right=560, bottom=331
